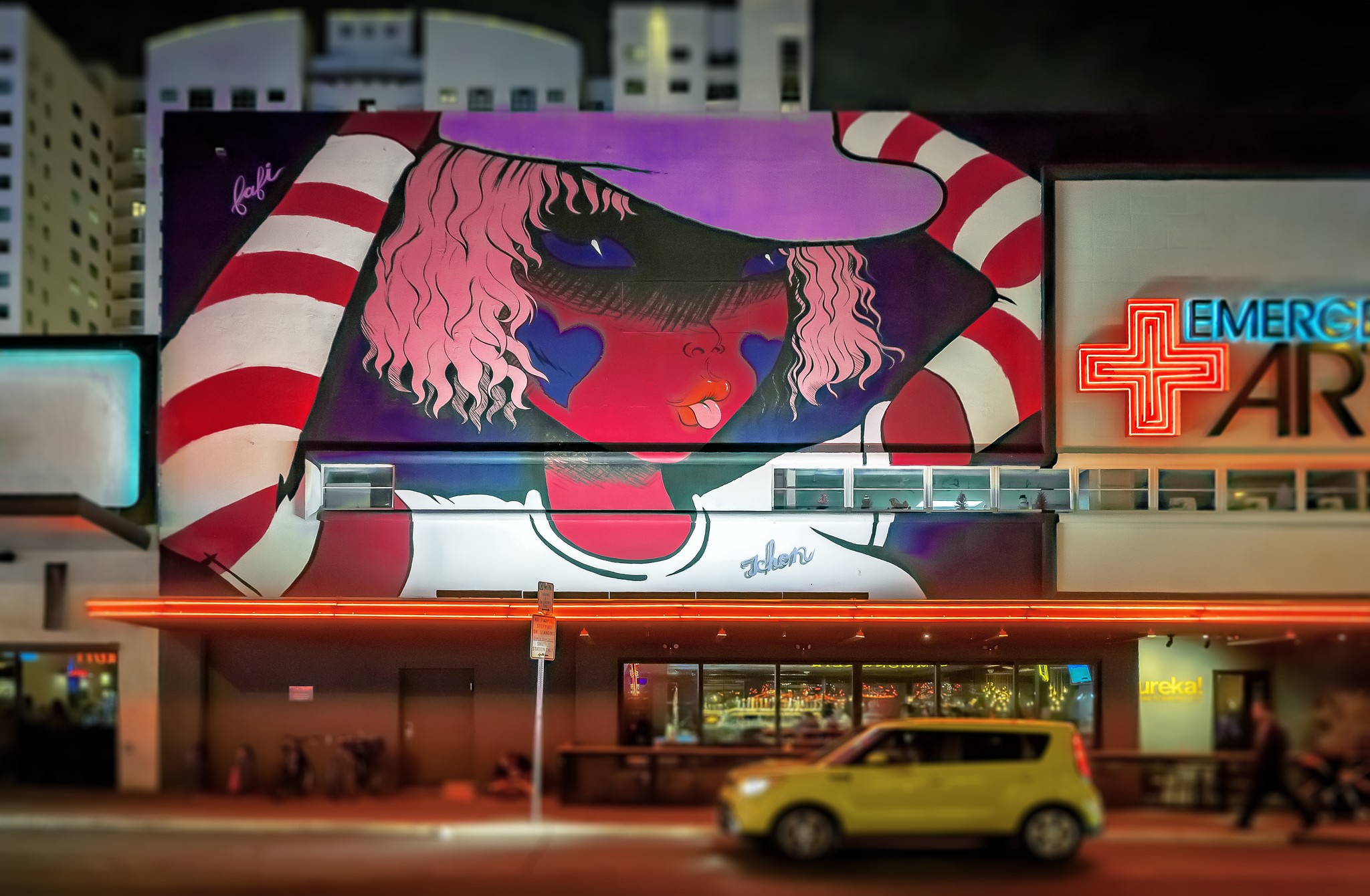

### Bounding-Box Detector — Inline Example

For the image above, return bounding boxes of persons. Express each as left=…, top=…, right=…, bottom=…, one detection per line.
left=1232, top=697, right=1305, bottom=826
left=796, top=711, right=819, bottom=728
left=1302, top=704, right=1353, bottom=825
left=822, top=707, right=836, bottom=728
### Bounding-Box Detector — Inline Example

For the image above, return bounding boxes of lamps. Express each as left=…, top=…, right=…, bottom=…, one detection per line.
left=580, top=628, right=588, bottom=636
left=857, top=627, right=865, bottom=637
left=998, top=628, right=1010, bottom=637
left=1165, top=635, right=1173, bottom=648
left=718, top=627, right=727, bottom=635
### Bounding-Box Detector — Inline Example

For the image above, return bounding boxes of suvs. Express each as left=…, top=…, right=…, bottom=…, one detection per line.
left=715, top=715, right=1107, bottom=874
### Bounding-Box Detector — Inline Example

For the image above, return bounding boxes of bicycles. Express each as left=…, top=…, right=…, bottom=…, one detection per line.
left=266, top=733, right=322, bottom=803
